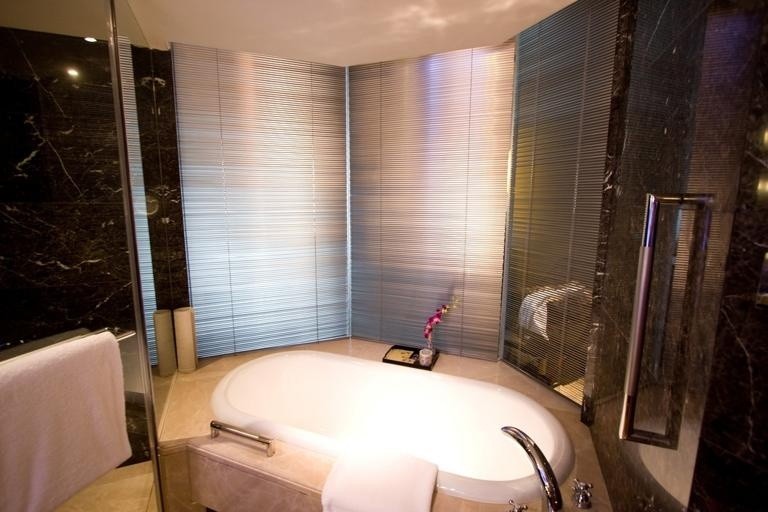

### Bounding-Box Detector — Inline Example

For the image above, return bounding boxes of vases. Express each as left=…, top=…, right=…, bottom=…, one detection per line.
left=172, top=305, right=199, bottom=374
left=151, top=308, right=177, bottom=379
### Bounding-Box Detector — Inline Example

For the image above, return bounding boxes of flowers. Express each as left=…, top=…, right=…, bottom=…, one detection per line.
left=423, top=287, right=464, bottom=346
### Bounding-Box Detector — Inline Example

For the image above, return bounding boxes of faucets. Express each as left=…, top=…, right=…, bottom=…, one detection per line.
left=501, top=426, right=564, bottom=511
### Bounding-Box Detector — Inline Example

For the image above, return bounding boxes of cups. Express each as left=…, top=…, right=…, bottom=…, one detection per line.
left=419, top=348, right=433, bottom=366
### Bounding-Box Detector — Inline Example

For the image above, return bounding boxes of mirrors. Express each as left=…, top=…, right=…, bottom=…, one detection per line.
left=112, top=0, right=177, bottom=445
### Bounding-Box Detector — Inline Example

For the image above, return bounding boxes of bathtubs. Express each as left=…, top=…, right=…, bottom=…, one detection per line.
left=210, top=350, right=575, bottom=505
